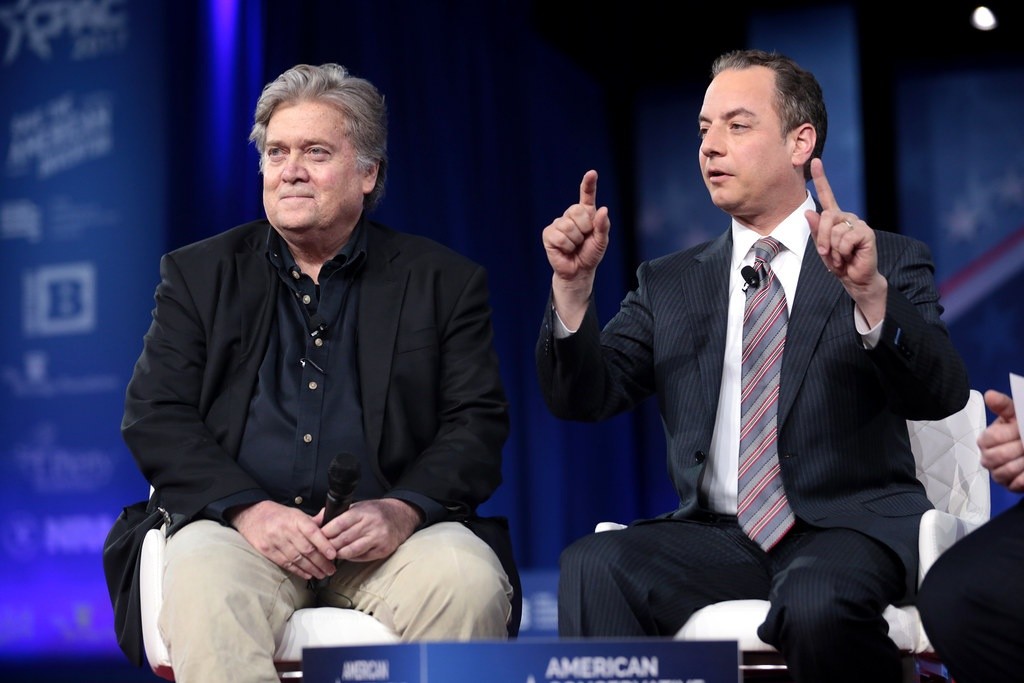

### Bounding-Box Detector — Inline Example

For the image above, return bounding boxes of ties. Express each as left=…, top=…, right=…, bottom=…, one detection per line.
left=736, top=235, right=794, bottom=552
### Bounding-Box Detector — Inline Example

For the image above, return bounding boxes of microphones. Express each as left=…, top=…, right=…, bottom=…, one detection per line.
left=309, top=451, right=362, bottom=590
left=310, top=314, right=327, bottom=333
left=741, top=266, right=760, bottom=288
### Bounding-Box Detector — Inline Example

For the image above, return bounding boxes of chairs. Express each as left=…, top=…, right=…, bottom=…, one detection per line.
left=101, top=484, right=523, bottom=683
left=594, top=390, right=991, bottom=674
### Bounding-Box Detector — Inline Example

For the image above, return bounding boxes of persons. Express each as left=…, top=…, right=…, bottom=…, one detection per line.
left=916, top=387, right=1023, bottom=683
left=532, top=47, right=972, bottom=683
left=116, top=55, right=517, bottom=683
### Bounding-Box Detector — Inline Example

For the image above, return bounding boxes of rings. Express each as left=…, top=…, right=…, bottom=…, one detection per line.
left=844, top=219, right=852, bottom=228
